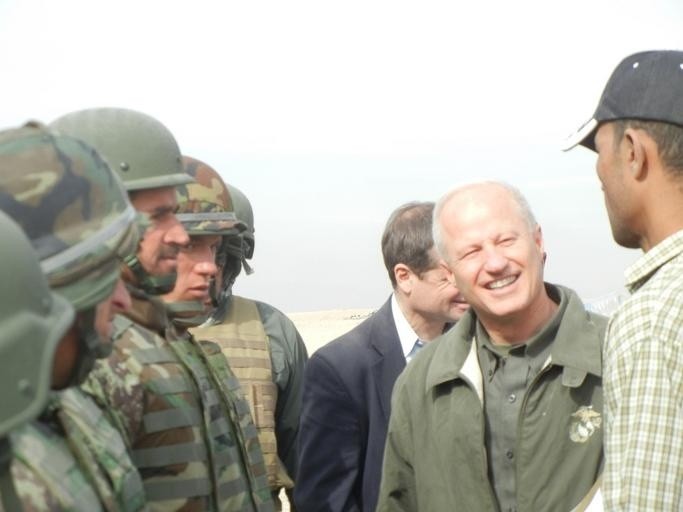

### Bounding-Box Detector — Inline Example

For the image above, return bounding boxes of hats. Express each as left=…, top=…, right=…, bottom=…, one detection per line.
left=557, top=47, right=683, bottom=152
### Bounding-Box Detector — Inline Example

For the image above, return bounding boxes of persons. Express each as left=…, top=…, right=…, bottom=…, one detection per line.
left=49, top=106, right=256, bottom=511
left=157, top=153, right=273, bottom=511
left=0, top=120, right=151, bottom=511
left=375, top=180, right=614, bottom=512
left=291, top=201, right=471, bottom=512
left=561, top=49, right=683, bottom=512
left=0, top=211, right=78, bottom=510
left=187, top=184, right=307, bottom=511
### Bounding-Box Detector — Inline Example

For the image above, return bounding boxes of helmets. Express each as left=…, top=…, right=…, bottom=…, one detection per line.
left=46, top=107, right=198, bottom=193
left=165, top=153, right=246, bottom=240
left=219, top=185, right=257, bottom=263
left=0, top=121, right=144, bottom=314
left=0, top=211, right=79, bottom=439
